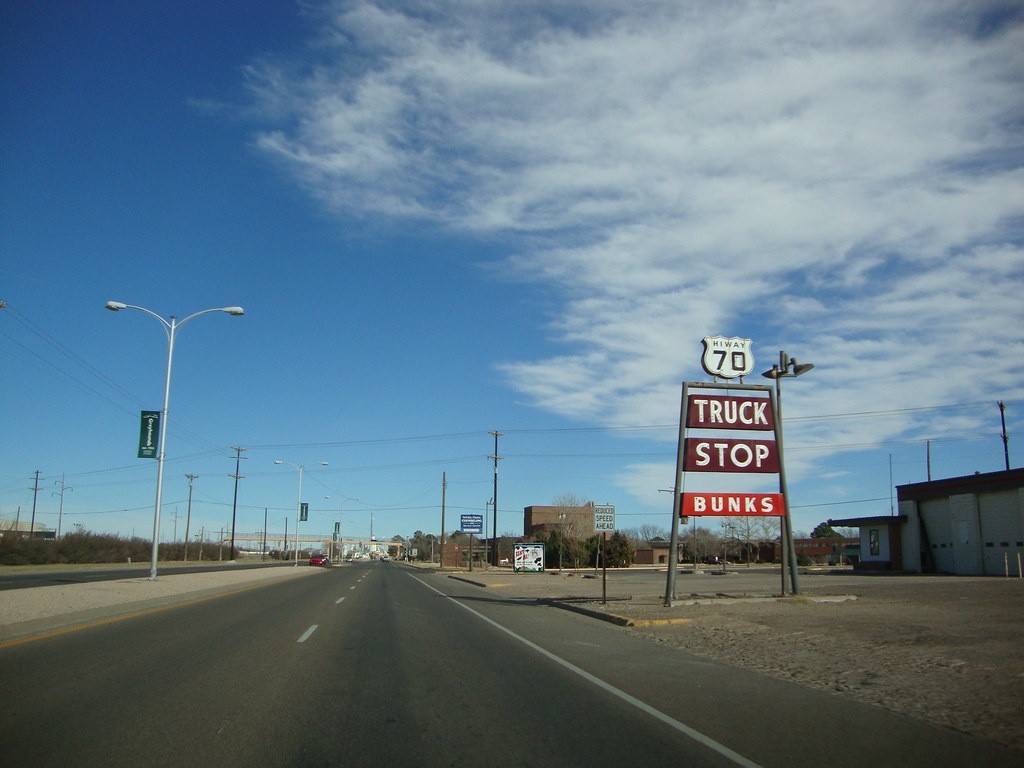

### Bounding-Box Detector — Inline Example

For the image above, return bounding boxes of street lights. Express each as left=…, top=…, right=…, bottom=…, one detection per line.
left=102, top=297, right=244, bottom=580
left=324, top=495, right=360, bottom=561
left=275, top=459, right=328, bottom=569
left=558, top=511, right=566, bottom=572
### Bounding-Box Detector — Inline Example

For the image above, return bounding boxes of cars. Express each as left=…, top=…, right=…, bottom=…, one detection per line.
left=309, top=553, right=327, bottom=566
left=383, top=556, right=388, bottom=562
left=346, top=556, right=353, bottom=562
left=500, top=557, right=509, bottom=565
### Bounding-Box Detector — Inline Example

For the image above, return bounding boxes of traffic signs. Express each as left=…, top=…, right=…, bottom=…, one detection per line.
left=460, top=514, right=483, bottom=534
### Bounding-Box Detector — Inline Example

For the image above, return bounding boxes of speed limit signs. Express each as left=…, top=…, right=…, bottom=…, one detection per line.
left=700, top=335, right=756, bottom=378
